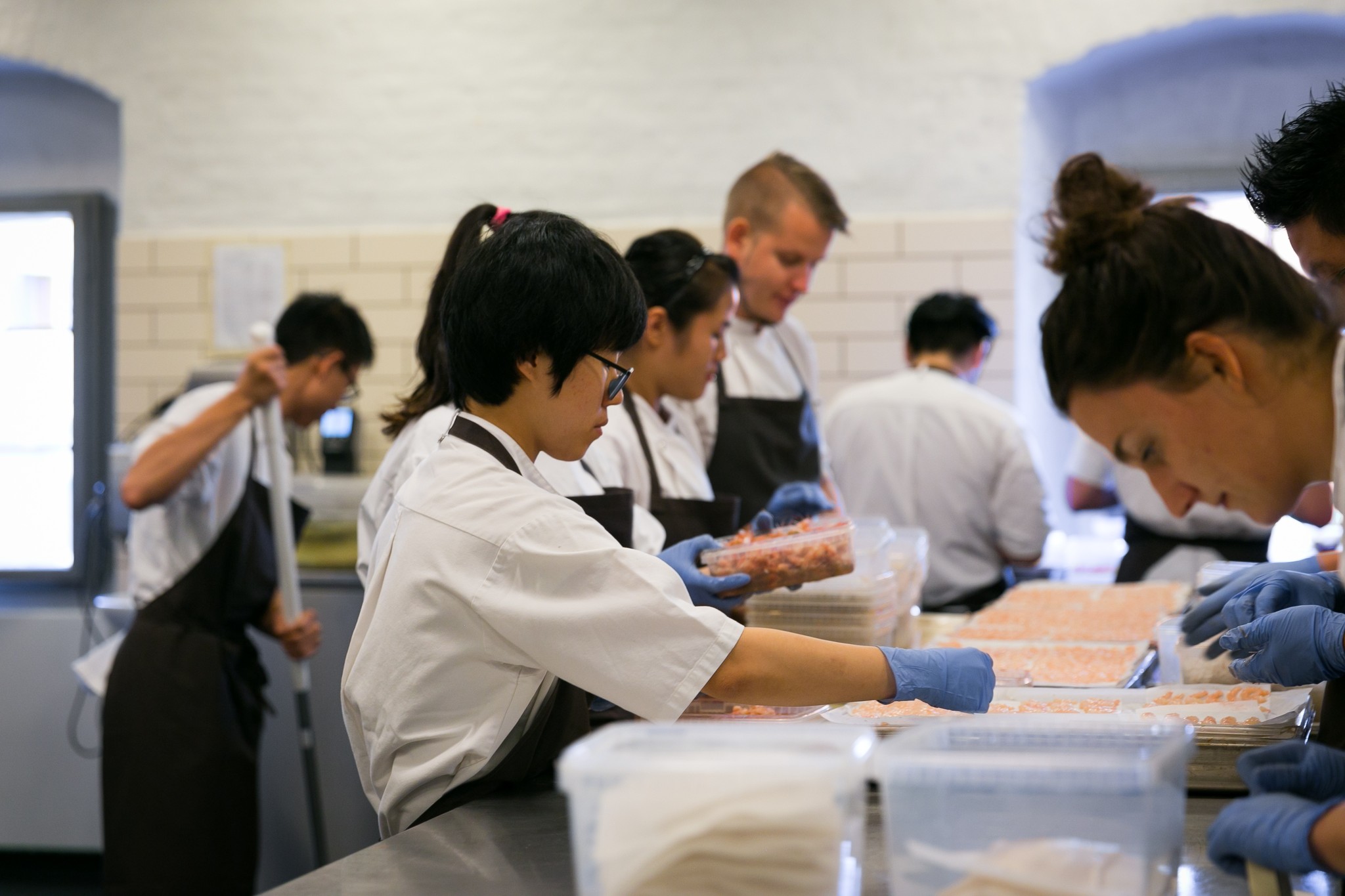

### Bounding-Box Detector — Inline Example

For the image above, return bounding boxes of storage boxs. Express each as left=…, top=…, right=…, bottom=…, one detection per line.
left=871, top=718, right=1196, bottom=894
left=553, top=720, right=870, bottom=894
left=700, top=520, right=854, bottom=598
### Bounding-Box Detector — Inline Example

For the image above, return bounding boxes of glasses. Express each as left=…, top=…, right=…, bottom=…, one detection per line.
left=584, top=348, right=635, bottom=402
left=340, top=362, right=363, bottom=402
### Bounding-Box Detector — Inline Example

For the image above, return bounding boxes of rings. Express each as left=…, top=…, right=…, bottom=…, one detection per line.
left=293, top=630, right=304, bottom=644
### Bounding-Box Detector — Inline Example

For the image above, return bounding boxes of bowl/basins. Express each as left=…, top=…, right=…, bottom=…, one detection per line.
left=551, top=509, right=1260, bottom=895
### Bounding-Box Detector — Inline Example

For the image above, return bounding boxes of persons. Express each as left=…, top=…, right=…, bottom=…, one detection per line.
left=581, top=227, right=835, bottom=543
left=815, top=291, right=1050, bottom=613
left=1173, top=83, right=1345, bottom=692
left=341, top=214, right=995, bottom=846
left=1065, top=425, right=1274, bottom=587
left=1034, top=150, right=1344, bottom=882
left=97, top=286, right=375, bottom=896
left=657, top=150, right=852, bottom=535
left=353, top=200, right=773, bottom=633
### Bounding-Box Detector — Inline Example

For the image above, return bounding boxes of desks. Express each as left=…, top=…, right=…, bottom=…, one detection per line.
left=263, top=518, right=1342, bottom=894
